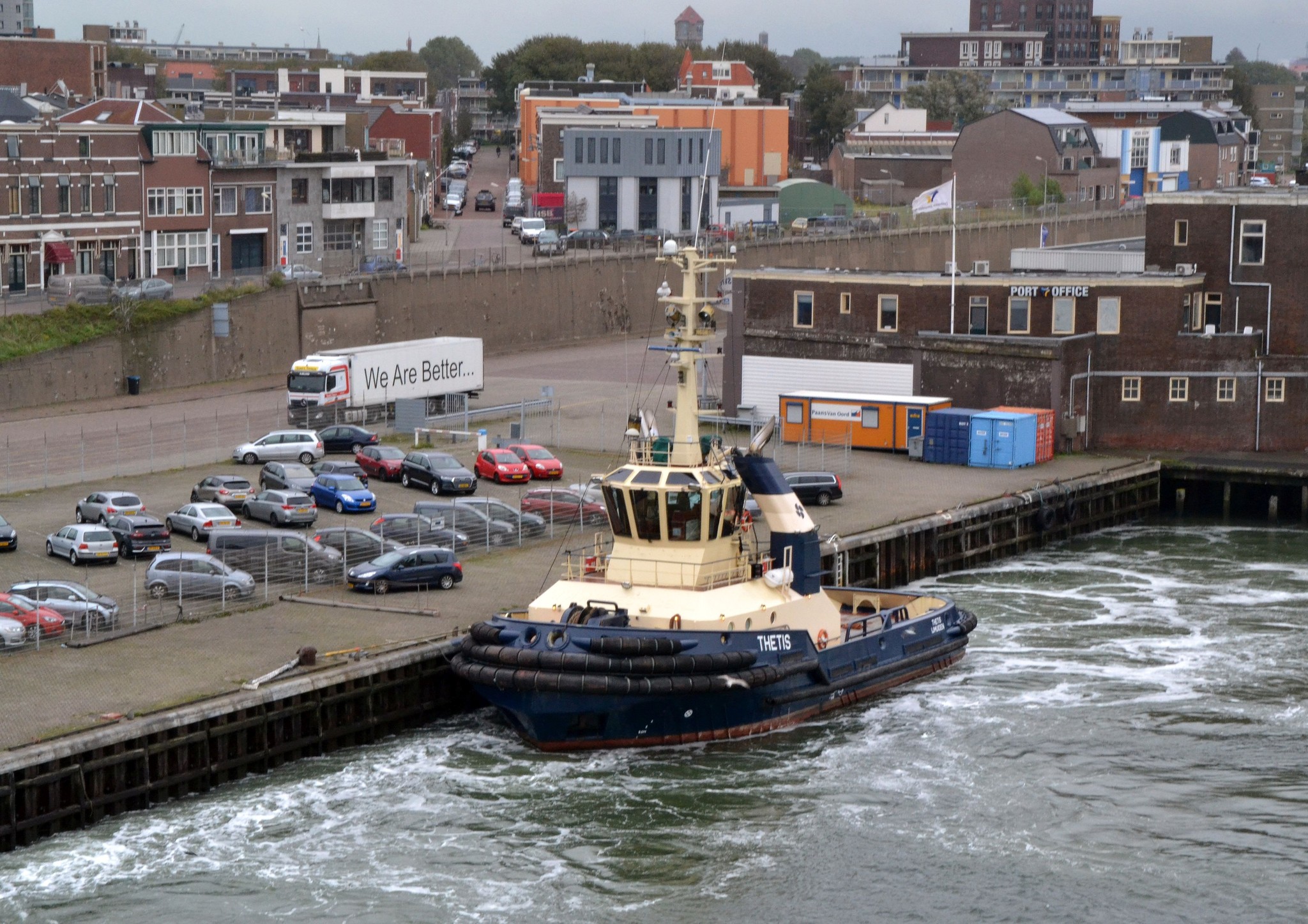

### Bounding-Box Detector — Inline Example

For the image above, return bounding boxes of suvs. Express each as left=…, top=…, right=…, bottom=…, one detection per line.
left=231, top=428, right=324, bottom=465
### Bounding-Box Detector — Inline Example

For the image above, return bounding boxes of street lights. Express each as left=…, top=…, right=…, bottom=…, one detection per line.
left=1034, top=154, right=1048, bottom=248
left=880, top=168, right=893, bottom=239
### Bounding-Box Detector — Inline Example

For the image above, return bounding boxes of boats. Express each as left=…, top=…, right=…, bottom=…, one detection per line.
left=444, top=236, right=981, bottom=755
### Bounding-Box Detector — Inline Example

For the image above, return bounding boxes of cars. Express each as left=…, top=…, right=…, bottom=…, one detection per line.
left=0, top=459, right=614, bottom=654
left=345, top=543, right=463, bottom=596
left=354, top=445, right=407, bottom=482
left=436, top=136, right=613, bottom=256
left=642, top=227, right=672, bottom=246
left=781, top=470, right=843, bottom=506
left=398, top=450, right=477, bottom=496
left=360, top=253, right=408, bottom=274
left=315, top=423, right=379, bottom=454
left=273, top=262, right=324, bottom=282
left=504, top=443, right=564, bottom=481
left=704, top=223, right=735, bottom=244
left=111, top=277, right=175, bottom=306
left=143, top=551, right=258, bottom=602
left=569, top=479, right=606, bottom=507
left=165, top=501, right=243, bottom=543
left=473, top=448, right=531, bottom=485
left=308, top=459, right=369, bottom=490
left=520, top=487, right=610, bottom=527
left=743, top=491, right=763, bottom=518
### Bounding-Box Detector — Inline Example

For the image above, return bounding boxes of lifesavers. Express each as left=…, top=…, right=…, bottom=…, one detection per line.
left=762, top=556, right=772, bottom=575
left=586, top=554, right=597, bottom=571
left=817, top=629, right=829, bottom=650
left=740, top=511, right=753, bottom=532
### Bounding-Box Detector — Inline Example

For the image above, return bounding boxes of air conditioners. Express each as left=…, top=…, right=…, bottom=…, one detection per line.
left=1176, top=264, right=1193, bottom=275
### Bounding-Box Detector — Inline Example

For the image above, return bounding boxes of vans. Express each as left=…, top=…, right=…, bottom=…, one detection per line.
left=44, top=272, right=121, bottom=310
left=742, top=210, right=900, bottom=241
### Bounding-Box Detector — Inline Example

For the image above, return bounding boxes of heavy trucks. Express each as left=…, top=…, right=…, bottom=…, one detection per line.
left=284, top=336, right=486, bottom=431
